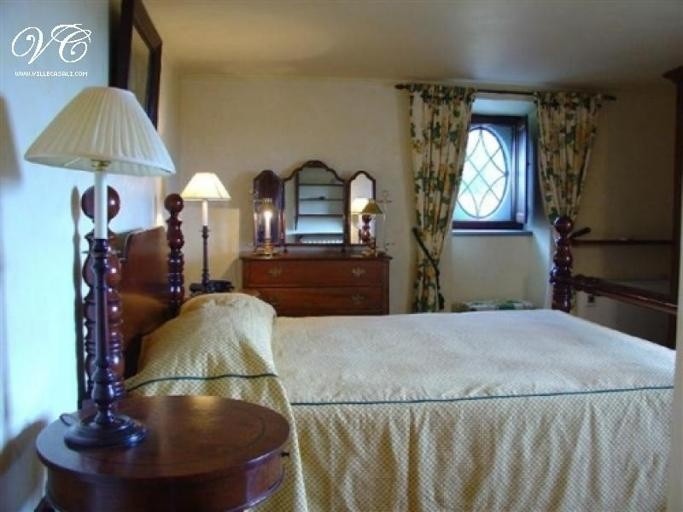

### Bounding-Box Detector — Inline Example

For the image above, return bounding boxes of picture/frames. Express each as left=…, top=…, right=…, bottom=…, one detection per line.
left=112, top=1, right=163, bottom=132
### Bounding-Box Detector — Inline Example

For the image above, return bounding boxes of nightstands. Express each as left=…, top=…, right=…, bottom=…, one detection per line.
left=34, top=394, right=291, bottom=512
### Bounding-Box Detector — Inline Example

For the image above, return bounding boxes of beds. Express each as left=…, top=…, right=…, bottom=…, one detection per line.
left=80, top=186, right=677, bottom=512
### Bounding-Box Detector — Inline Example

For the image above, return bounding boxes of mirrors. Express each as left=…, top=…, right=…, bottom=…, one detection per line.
left=282, top=159, right=377, bottom=247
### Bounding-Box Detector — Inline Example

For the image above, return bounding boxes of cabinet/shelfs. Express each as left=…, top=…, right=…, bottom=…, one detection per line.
left=239, top=253, right=392, bottom=316
left=294, top=171, right=344, bottom=231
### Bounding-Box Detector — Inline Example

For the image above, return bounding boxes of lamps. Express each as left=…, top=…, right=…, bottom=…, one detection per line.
left=22, top=85, right=177, bottom=452
left=254, top=169, right=280, bottom=256
left=179, top=173, right=232, bottom=293
left=361, top=198, right=384, bottom=255
